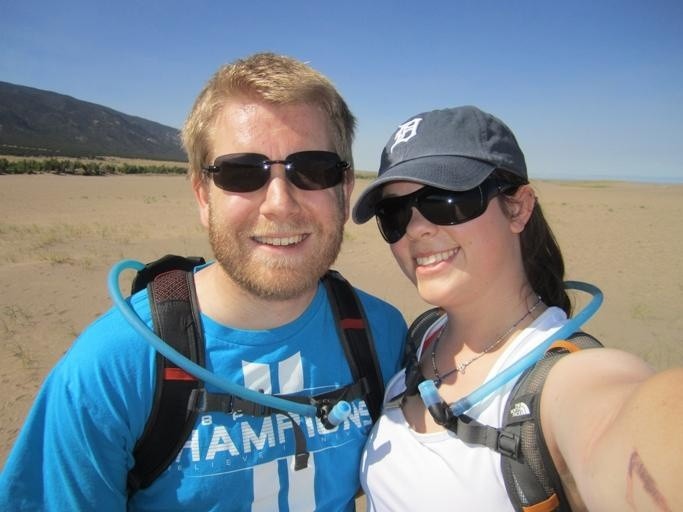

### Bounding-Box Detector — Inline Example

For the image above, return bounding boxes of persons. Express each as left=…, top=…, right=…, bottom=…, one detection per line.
left=350, top=106, right=682, bottom=511
left=2, top=54, right=410, bottom=512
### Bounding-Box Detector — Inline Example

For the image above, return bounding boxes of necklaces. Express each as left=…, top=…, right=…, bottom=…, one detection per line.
left=430, top=295, right=541, bottom=388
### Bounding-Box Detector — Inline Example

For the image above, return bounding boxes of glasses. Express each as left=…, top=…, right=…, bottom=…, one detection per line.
left=376, top=183, right=502, bottom=243
left=201, top=150, right=351, bottom=192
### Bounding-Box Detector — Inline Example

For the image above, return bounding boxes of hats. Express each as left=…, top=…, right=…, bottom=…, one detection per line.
left=352, top=104, right=528, bottom=225
